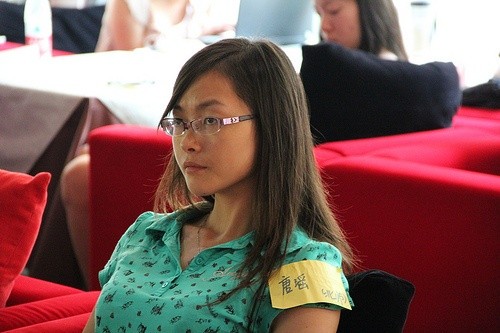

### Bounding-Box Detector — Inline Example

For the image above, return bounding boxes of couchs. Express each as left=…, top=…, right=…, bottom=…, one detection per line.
left=0, top=106, right=500, bottom=333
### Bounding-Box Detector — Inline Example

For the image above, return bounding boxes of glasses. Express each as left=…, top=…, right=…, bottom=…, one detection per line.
left=160, top=113, right=257, bottom=137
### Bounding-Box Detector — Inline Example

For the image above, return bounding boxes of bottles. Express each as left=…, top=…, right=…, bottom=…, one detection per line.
left=24, top=0, right=53, bottom=80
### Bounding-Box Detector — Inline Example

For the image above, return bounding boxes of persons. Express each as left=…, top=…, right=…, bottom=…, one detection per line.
left=314, top=0, right=409, bottom=62
left=81, top=38, right=361, bottom=333
left=61, top=1, right=235, bottom=290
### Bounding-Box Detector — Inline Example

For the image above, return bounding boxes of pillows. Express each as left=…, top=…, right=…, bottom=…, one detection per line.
left=299, top=41, right=461, bottom=147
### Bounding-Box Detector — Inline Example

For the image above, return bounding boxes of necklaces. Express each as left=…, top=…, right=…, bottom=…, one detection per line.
left=198, top=216, right=208, bottom=252
